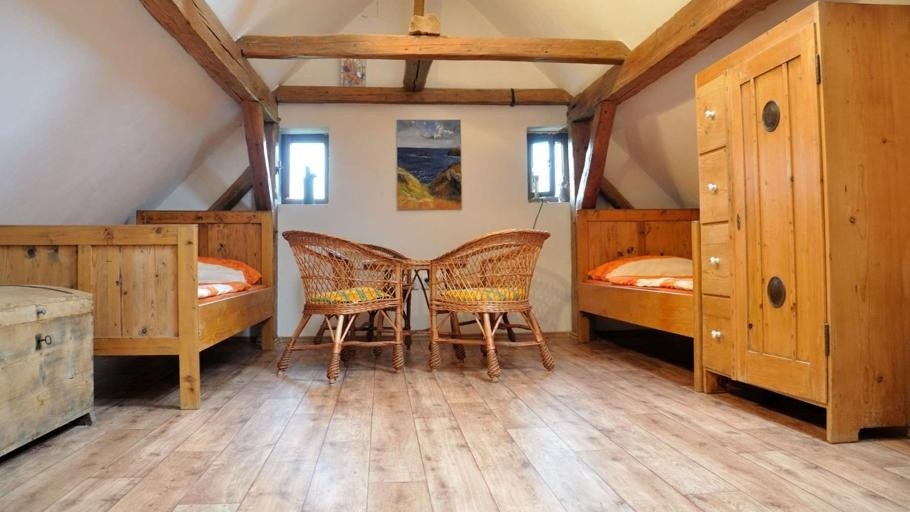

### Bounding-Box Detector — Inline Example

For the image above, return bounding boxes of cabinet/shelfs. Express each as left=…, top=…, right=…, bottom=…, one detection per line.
left=694, top=1, right=910, bottom=443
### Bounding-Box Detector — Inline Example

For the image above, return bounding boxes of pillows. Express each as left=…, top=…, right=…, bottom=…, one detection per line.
left=587, top=256, right=693, bottom=286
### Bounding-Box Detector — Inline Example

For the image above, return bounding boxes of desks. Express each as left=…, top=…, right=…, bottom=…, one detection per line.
left=363, top=255, right=469, bottom=361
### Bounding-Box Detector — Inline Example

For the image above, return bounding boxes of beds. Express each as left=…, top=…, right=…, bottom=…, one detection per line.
left=1, top=211, right=277, bottom=409
left=571, top=209, right=702, bottom=393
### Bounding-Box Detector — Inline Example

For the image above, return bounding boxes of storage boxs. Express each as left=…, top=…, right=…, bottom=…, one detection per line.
left=1, top=286, right=95, bottom=462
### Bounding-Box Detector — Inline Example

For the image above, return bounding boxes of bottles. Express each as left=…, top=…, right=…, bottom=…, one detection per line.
left=303, top=167, right=315, bottom=203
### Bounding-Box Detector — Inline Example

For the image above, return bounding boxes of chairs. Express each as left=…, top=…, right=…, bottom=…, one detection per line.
left=427, top=231, right=555, bottom=382
left=277, top=230, right=415, bottom=384
